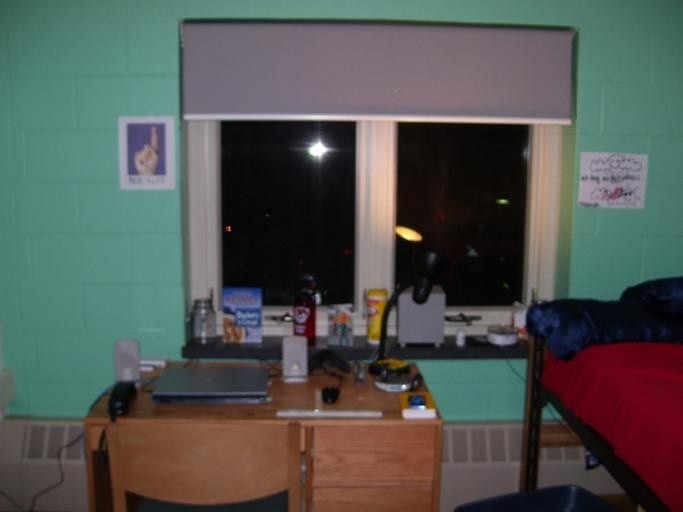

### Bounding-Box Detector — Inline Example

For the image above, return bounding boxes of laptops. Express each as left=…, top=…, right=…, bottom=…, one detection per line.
left=152, top=368, right=270, bottom=404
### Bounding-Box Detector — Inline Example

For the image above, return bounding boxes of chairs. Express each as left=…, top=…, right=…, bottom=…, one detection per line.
left=106, top=419, right=302, bottom=512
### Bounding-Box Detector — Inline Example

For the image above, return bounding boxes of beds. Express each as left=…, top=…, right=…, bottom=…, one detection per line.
left=523, top=314, right=680, bottom=511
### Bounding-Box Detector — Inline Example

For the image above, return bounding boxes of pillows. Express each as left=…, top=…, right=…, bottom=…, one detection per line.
left=523, top=284, right=683, bottom=364
left=619, top=275, right=682, bottom=346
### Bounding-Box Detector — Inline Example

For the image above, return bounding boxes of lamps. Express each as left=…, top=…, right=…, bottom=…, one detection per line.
left=366, top=216, right=440, bottom=394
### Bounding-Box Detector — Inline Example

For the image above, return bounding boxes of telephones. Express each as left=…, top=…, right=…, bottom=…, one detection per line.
left=110, top=381, right=137, bottom=419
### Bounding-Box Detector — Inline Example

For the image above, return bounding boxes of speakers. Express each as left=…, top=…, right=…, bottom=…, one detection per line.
left=279, top=336, right=310, bottom=383
left=114, top=339, right=140, bottom=381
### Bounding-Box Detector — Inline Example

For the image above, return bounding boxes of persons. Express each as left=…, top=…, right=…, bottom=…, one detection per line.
left=128, top=125, right=165, bottom=174
left=330, top=305, right=353, bottom=349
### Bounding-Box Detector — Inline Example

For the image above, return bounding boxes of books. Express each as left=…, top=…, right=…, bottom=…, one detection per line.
left=398, top=390, right=436, bottom=420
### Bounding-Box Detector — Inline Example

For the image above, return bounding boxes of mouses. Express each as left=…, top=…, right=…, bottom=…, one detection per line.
left=322, top=385, right=339, bottom=403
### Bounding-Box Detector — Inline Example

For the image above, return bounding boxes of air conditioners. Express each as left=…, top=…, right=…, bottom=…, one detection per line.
left=0, top=413, right=89, bottom=512
left=441, top=414, right=640, bottom=512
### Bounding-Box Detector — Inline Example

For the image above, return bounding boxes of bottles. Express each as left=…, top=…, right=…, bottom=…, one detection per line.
left=191, top=299, right=216, bottom=347
left=292, top=280, right=316, bottom=345
left=366, top=288, right=387, bottom=345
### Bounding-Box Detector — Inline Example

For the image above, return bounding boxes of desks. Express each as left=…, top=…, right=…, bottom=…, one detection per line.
left=80, top=354, right=441, bottom=511
